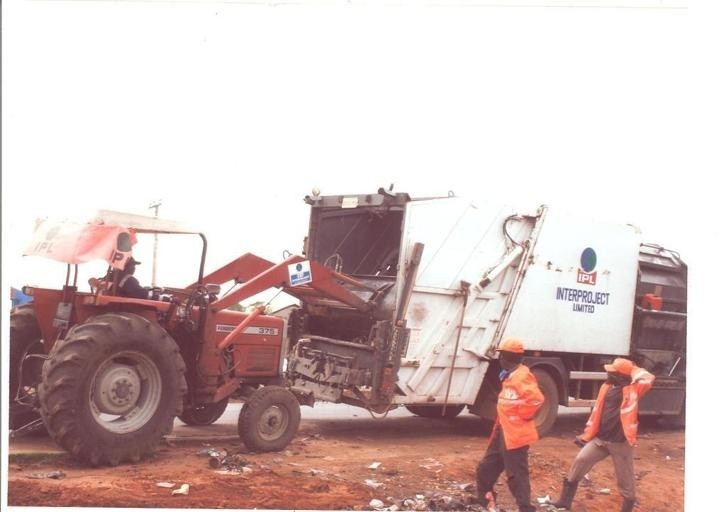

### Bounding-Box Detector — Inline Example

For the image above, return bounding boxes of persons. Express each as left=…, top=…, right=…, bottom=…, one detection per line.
left=545, top=356, right=657, bottom=511
left=102, top=255, right=147, bottom=299
left=474, top=338, right=545, bottom=511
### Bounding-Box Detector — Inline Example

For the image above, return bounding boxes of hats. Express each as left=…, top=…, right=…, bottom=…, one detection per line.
left=603, top=356, right=634, bottom=377
left=126, top=256, right=141, bottom=265
left=494, top=338, right=524, bottom=354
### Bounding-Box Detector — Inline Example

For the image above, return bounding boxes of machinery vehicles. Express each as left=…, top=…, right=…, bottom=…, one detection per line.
left=8, top=218, right=425, bottom=473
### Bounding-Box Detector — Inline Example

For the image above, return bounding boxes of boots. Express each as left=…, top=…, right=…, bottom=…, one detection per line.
left=545, top=477, right=578, bottom=510
left=620, top=498, right=634, bottom=512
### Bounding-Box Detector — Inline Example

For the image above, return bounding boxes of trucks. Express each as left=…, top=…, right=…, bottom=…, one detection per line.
left=280, top=183, right=687, bottom=443
